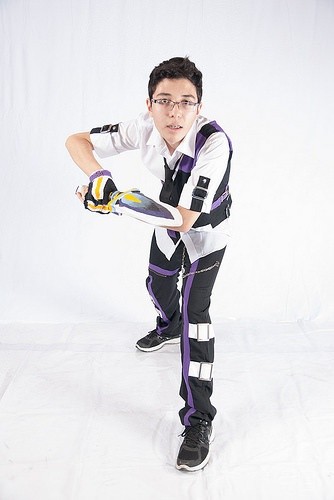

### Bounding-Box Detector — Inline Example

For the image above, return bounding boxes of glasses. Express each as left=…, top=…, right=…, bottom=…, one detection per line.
left=151, top=98, right=200, bottom=111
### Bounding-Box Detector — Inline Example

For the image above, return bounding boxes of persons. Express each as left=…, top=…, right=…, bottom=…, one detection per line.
left=66, top=57, right=233, bottom=471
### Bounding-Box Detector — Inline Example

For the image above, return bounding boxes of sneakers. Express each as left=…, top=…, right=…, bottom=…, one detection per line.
left=176, top=423, right=216, bottom=472
left=135, top=329, right=181, bottom=352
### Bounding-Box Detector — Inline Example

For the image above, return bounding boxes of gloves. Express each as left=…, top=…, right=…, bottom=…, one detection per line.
left=84, top=170, right=118, bottom=214
